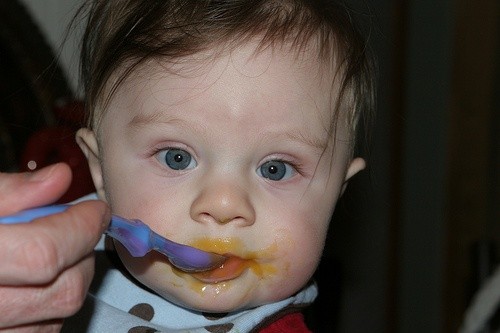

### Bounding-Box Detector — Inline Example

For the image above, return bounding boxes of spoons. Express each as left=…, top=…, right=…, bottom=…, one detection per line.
left=0, top=205, right=227, bottom=272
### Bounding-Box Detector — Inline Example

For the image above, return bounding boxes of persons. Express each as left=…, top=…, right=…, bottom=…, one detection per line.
left=76, top=0, right=376, bottom=333
